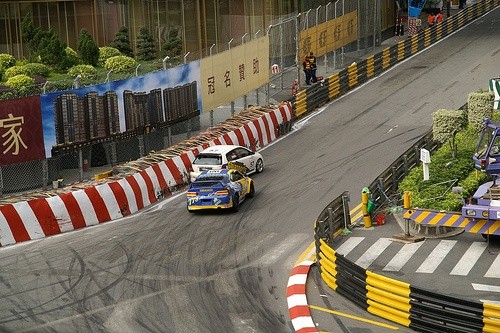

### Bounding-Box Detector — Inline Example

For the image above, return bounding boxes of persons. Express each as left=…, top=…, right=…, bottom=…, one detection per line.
left=303, top=56, right=312, bottom=85
left=435, top=11, right=444, bottom=23
left=309, top=52, right=317, bottom=82
left=428, top=12, right=435, bottom=27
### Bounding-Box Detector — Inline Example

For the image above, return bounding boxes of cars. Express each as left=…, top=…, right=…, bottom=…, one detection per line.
left=190, top=144, right=265, bottom=185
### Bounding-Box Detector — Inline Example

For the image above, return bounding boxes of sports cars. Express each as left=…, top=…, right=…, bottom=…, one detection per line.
left=185, top=168, right=255, bottom=214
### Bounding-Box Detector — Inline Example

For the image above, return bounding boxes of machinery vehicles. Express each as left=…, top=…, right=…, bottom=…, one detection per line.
left=391, top=120, right=500, bottom=255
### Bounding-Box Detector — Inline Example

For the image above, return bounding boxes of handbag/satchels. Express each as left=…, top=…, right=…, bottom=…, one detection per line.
left=310, top=63, right=317, bottom=71
